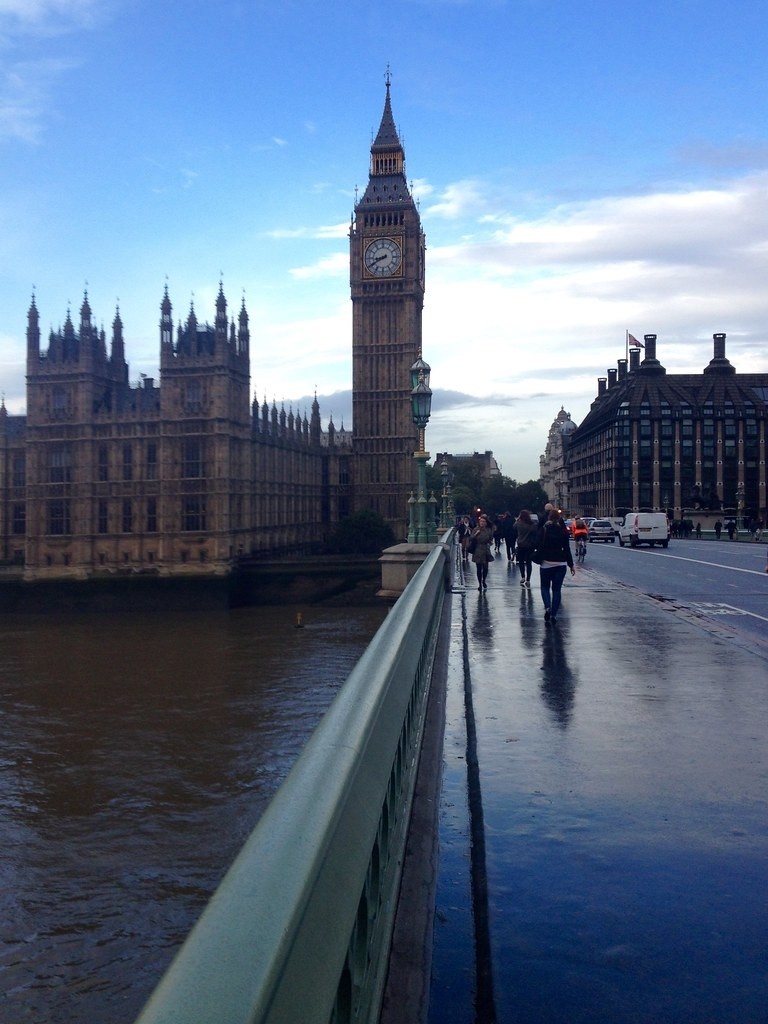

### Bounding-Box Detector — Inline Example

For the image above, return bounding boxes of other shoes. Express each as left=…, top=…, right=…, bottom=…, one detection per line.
left=525, top=582, right=530, bottom=587
left=575, top=553, right=578, bottom=556
left=482, top=582, right=487, bottom=588
left=520, top=579, right=525, bottom=583
left=584, top=549, right=586, bottom=554
left=544, top=608, right=551, bottom=621
left=478, top=587, right=483, bottom=590
left=552, top=618, right=557, bottom=624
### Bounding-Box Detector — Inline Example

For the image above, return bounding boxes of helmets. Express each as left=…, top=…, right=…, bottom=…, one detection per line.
left=575, top=515, right=580, bottom=519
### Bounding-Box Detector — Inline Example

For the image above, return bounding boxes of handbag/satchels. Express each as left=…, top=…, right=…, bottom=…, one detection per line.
left=466, top=542, right=475, bottom=554
left=530, top=527, right=547, bottom=565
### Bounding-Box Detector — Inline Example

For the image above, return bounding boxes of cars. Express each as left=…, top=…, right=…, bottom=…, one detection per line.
left=563, top=516, right=615, bottom=543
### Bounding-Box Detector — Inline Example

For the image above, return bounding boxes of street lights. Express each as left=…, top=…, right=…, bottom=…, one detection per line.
left=403, top=346, right=440, bottom=545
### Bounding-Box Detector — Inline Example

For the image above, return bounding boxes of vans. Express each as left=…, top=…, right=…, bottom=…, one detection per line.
left=618, top=512, right=671, bottom=548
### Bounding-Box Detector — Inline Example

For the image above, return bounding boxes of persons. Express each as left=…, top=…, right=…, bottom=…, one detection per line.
left=749, top=517, right=765, bottom=542
left=537, top=509, right=575, bottom=623
left=458, top=512, right=517, bottom=562
left=571, top=514, right=589, bottom=556
left=669, top=520, right=694, bottom=539
left=714, top=520, right=722, bottom=539
left=470, top=515, right=493, bottom=592
left=538, top=503, right=566, bottom=530
left=512, top=510, right=536, bottom=589
left=695, top=523, right=702, bottom=539
left=724, top=519, right=738, bottom=540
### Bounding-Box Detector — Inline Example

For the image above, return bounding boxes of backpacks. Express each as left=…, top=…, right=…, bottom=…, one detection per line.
left=576, top=520, right=584, bottom=529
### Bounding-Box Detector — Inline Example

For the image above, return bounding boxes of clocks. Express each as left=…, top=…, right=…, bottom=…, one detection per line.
left=364, top=236, right=402, bottom=278
